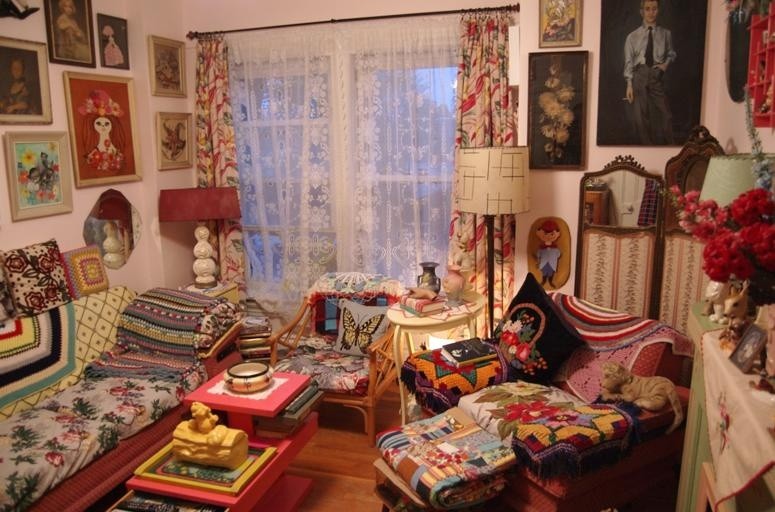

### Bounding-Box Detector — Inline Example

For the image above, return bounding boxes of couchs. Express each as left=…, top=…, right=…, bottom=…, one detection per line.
left=401, top=292, right=696, bottom=512
left=0, top=286, right=244, bottom=512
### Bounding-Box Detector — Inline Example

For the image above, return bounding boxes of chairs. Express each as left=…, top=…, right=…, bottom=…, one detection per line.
left=264, top=272, right=405, bottom=448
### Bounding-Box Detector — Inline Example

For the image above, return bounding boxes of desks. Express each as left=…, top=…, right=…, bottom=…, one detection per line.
left=181, top=280, right=239, bottom=305
left=674, top=302, right=775, bottom=512
left=386, top=290, right=485, bottom=426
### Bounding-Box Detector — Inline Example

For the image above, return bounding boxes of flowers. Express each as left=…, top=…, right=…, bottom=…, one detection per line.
left=659, top=82, right=775, bottom=285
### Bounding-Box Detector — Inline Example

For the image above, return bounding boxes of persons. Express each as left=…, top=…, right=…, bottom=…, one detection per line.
left=621, top=2, right=682, bottom=147
left=52, top=0, right=89, bottom=62
left=0, top=58, right=33, bottom=114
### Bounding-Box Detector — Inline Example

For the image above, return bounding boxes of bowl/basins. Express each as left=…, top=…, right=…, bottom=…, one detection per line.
left=222, top=360, right=274, bottom=394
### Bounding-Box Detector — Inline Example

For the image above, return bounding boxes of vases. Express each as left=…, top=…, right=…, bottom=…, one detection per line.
left=751, top=286, right=775, bottom=306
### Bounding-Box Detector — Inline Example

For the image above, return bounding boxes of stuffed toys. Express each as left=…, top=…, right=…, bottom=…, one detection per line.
left=599, top=359, right=685, bottom=437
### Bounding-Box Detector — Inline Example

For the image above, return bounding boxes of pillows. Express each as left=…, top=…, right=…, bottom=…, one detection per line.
left=332, top=298, right=392, bottom=358
left=493, top=272, right=587, bottom=387
left=0, top=238, right=110, bottom=319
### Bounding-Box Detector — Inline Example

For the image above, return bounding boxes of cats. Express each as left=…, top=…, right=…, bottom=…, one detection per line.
left=598, top=360, right=683, bottom=417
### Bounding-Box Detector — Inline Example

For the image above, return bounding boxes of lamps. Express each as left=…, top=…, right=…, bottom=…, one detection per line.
left=159, top=186, right=242, bottom=289
left=453, top=146, right=531, bottom=339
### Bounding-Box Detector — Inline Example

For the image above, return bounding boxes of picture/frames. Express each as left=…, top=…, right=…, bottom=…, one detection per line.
left=728, top=323, right=768, bottom=375
left=527, top=50, right=590, bottom=171
left=539, top=0, right=584, bottom=49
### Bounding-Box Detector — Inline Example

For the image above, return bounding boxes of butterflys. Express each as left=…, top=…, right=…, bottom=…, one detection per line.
left=339, top=305, right=385, bottom=354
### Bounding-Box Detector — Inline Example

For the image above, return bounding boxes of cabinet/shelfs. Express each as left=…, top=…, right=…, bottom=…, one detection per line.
left=743, top=1, right=775, bottom=128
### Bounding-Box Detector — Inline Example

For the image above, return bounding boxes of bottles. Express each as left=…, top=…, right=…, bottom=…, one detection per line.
left=415, top=262, right=441, bottom=296
left=443, top=265, right=467, bottom=303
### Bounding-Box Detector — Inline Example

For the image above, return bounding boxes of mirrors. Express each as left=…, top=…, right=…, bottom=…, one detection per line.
left=574, top=154, right=664, bottom=320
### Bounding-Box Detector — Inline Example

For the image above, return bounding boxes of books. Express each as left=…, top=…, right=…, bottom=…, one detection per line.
left=401, top=293, right=447, bottom=313
left=236, top=297, right=273, bottom=364
left=399, top=304, right=443, bottom=318
